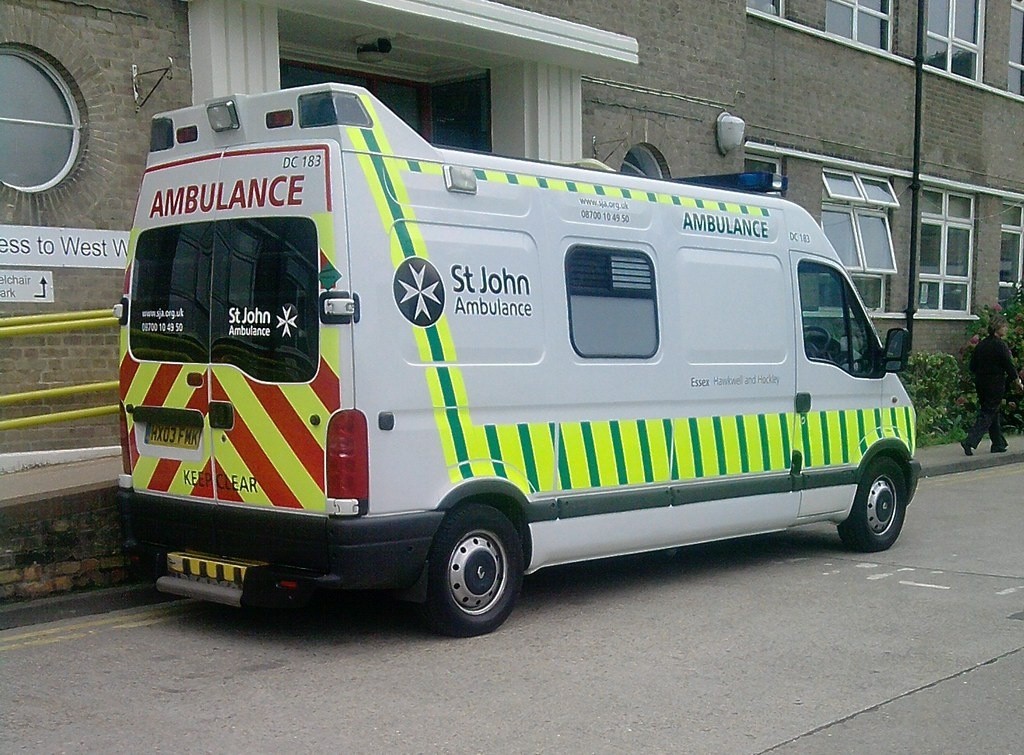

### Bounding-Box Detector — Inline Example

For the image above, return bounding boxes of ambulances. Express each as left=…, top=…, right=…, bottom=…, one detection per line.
left=108, top=80, right=925, bottom=637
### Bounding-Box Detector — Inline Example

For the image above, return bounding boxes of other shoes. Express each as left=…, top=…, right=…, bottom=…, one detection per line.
left=961, top=440, right=973, bottom=456
left=990, top=443, right=1010, bottom=453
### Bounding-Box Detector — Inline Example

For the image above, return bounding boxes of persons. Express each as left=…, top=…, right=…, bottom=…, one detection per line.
left=960, top=315, right=1024, bottom=457
